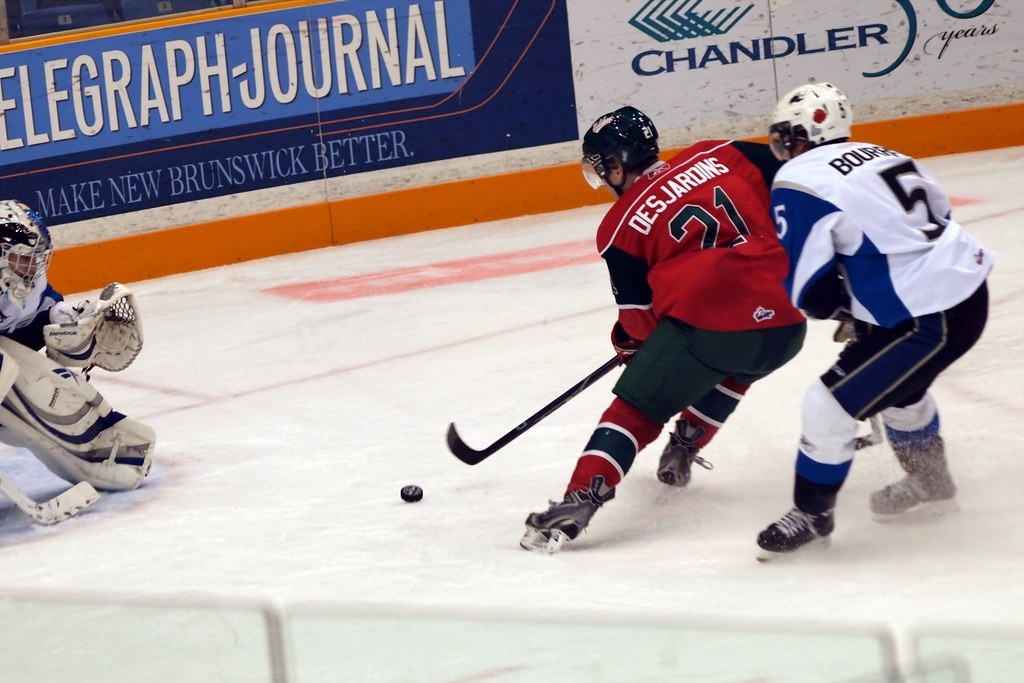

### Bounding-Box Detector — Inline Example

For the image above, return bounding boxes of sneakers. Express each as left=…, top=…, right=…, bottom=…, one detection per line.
left=870, top=467, right=956, bottom=523
left=657, top=417, right=714, bottom=496
left=756, top=505, right=836, bottom=563
left=520, top=475, right=616, bottom=554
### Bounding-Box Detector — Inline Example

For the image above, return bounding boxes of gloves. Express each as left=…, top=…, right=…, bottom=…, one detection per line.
left=609, top=321, right=641, bottom=365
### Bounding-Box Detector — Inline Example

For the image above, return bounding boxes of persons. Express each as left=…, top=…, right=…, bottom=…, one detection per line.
left=756, top=82, right=998, bottom=562
left=520, top=106, right=809, bottom=554
left=0, top=196, right=155, bottom=495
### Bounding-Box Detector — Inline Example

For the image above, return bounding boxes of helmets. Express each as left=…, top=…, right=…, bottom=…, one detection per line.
left=581, top=106, right=658, bottom=190
left=0, top=199, right=51, bottom=309
left=768, top=82, right=852, bottom=161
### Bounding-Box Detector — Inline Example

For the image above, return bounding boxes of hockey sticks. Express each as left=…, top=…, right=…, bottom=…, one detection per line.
left=445, top=354, right=625, bottom=467
left=843, top=322, right=883, bottom=449
left=0, top=472, right=101, bottom=525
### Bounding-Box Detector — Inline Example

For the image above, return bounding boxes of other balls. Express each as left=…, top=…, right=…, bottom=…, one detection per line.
left=402, top=484, right=424, bottom=502
